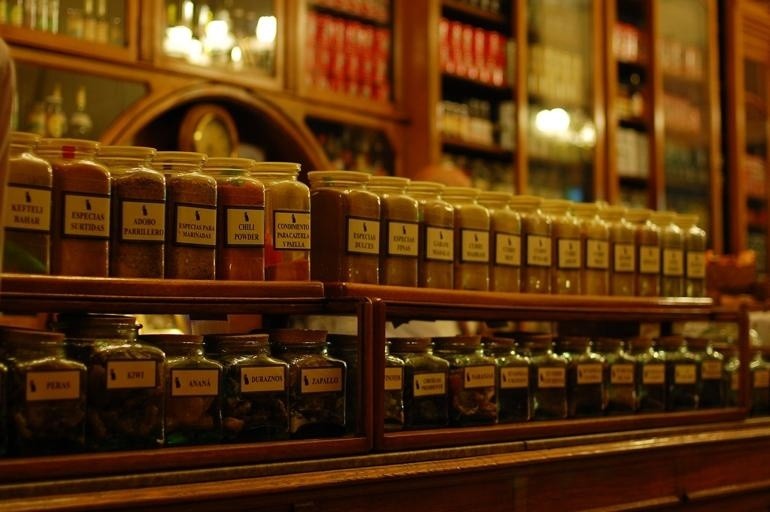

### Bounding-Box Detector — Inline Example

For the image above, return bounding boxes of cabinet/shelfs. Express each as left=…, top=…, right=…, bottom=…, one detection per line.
left=42, top=81, right=71, bottom=140
left=0, top=1, right=770, bottom=512
left=163, top=0, right=275, bottom=77
left=68, top=83, right=96, bottom=140
left=22, top=98, right=45, bottom=137
left=0, top=0, right=125, bottom=49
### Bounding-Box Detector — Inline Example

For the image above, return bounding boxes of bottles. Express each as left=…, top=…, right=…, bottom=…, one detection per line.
left=163, top=0, right=275, bottom=77
left=42, top=81, right=71, bottom=140
left=0, top=0, right=125, bottom=49
left=3, top=308, right=353, bottom=449
left=68, top=83, right=96, bottom=140
left=307, top=169, right=707, bottom=300
left=2, top=131, right=312, bottom=281
left=381, top=335, right=742, bottom=430
left=300, top=1, right=711, bottom=213
left=22, top=98, right=45, bottom=137
